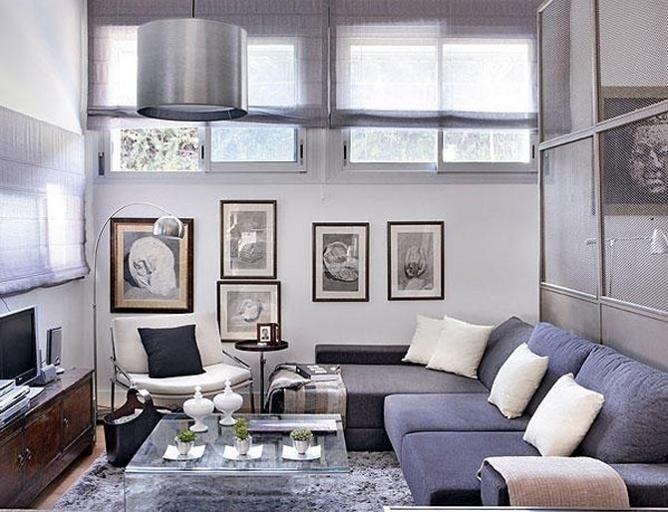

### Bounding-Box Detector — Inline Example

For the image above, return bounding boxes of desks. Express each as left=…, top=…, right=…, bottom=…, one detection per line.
left=233, top=337, right=287, bottom=413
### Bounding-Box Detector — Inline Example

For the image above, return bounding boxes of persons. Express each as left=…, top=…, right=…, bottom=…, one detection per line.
left=621, top=112, right=668, bottom=197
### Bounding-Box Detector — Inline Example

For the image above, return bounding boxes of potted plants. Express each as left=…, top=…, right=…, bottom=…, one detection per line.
left=233, top=417, right=252, bottom=455
left=289, top=427, right=313, bottom=454
left=174, top=430, right=197, bottom=454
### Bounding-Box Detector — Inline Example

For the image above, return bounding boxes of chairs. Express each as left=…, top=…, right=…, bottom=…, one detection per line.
left=104, top=309, right=258, bottom=414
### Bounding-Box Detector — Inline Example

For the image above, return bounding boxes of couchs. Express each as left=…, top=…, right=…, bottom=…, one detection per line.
left=268, top=313, right=666, bottom=509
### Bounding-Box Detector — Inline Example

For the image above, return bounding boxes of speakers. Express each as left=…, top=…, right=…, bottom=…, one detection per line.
left=46, top=326, right=64, bottom=374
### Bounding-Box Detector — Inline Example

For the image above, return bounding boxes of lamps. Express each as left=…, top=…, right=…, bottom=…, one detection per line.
left=88, top=198, right=183, bottom=422
left=585, top=229, right=668, bottom=298
left=136, top=0, right=247, bottom=121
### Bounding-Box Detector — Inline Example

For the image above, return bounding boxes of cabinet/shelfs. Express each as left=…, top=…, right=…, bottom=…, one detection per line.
left=61, top=368, right=95, bottom=470
left=0, top=395, right=61, bottom=512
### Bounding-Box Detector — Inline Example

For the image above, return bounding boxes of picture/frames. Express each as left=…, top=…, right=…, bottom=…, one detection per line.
left=311, top=220, right=370, bottom=302
left=593, top=84, right=667, bottom=217
left=212, top=279, right=281, bottom=344
left=220, top=198, right=276, bottom=278
left=107, top=216, right=195, bottom=314
left=256, top=322, right=272, bottom=342
left=387, top=220, right=445, bottom=300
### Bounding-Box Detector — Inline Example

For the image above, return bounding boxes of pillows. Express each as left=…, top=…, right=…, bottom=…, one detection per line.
left=425, top=315, right=496, bottom=379
left=523, top=367, right=604, bottom=457
left=486, top=339, right=548, bottom=418
left=136, top=322, right=207, bottom=378
left=401, top=312, right=444, bottom=362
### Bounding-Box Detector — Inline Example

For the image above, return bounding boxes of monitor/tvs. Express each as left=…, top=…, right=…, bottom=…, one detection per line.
left=0, top=304, right=45, bottom=400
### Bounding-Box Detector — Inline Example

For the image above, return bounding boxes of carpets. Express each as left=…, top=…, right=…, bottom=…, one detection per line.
left=44, top=447, right=416, bottom=511
